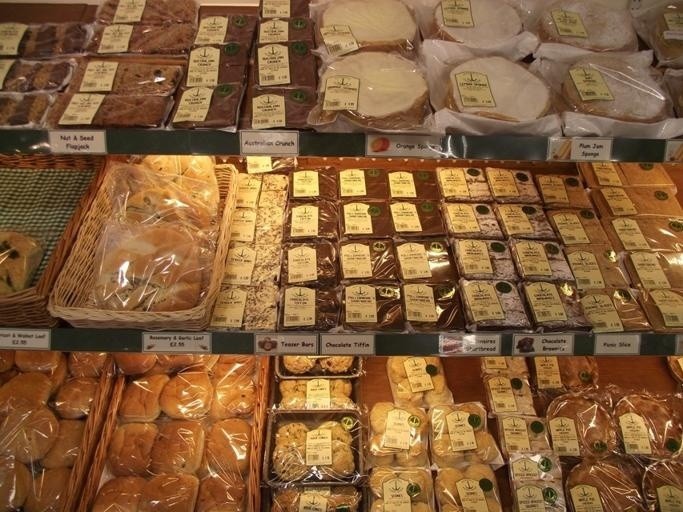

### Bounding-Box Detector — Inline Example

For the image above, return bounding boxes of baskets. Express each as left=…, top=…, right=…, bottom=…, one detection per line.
left=76, top=355, right=270, bottom=510
left=46, top=161, right=238, bottom=333
left=0, top=152, right=112, bottom=328
left=0, top=348, right=115, bottom=512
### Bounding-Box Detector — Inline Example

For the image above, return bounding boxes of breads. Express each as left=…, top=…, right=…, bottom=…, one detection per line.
left=0, top=0, right=683, bottom=129
left=0, top=348, right=683, bottom=512
left=94, top=155, right=683, bottom=332
left=0, top=231, right=44, bottom=297
